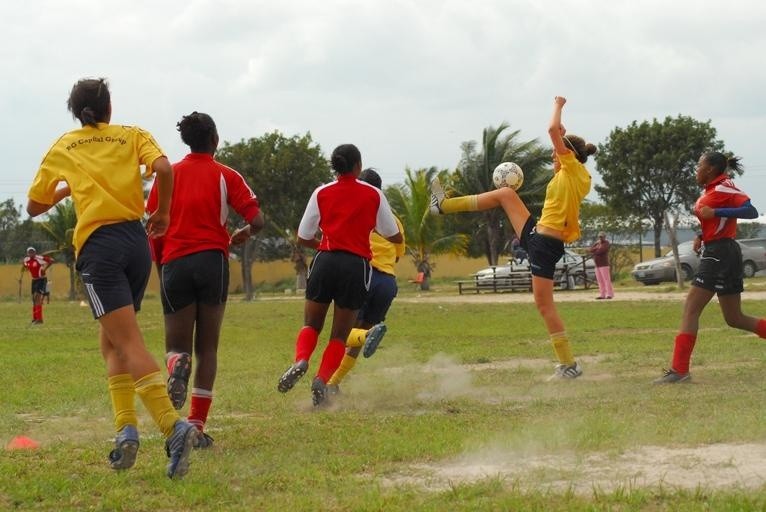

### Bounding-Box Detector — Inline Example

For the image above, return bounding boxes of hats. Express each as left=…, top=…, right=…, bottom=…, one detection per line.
left=598, top=232, right=606, bottom=237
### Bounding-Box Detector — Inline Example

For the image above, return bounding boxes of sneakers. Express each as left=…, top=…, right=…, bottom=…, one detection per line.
left=428, top=174, right=447, bottom=217
left=363, top=323, right=387, bottom=358
left=548, top=361, right=582, bottom=381
left=109, top=431, right=140, bottom=471
left=192, top=432, right=216, bottom=450
left=164, top=351, right=193, bottom=410
left=325, top=384, right=340, bottom=395
left=310, top=380, right=325, bottom=407
left=277, top=361, right=308, bottom=394
left=165, top=421, right=196, bottom=479
left=30, top=318, right=44, bottom=326
left=654, top=371, right=690, bottom=386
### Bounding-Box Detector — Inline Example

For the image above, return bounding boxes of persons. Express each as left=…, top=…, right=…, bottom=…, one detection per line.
left=322, top=167, right=406, bottom=397
left=652, top=149, right=766, bottom=383
left=589, top=229, right=616, bottom=302
left=18, top=247, right=55, bottom=325
left=26, top=78, right=198, bottom=481
left=427, top=95, right=600, bottom=385
left=144, top=111, right=264, bottom=450
left=277, top=142, right=404, bottom=406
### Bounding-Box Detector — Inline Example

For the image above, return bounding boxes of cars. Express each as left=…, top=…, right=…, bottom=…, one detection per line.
left=633, top=240, right=766, bottom=285
left=476, top=250, right=597, bottom=288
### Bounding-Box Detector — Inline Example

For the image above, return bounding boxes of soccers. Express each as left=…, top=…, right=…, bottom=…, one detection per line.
left=492, top=162, right=525, bottom=191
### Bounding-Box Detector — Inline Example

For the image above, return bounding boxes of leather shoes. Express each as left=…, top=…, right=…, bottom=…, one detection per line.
left=607, top=295, right=613, bottom=300
left=596, top=296, right=605, bottom=300
